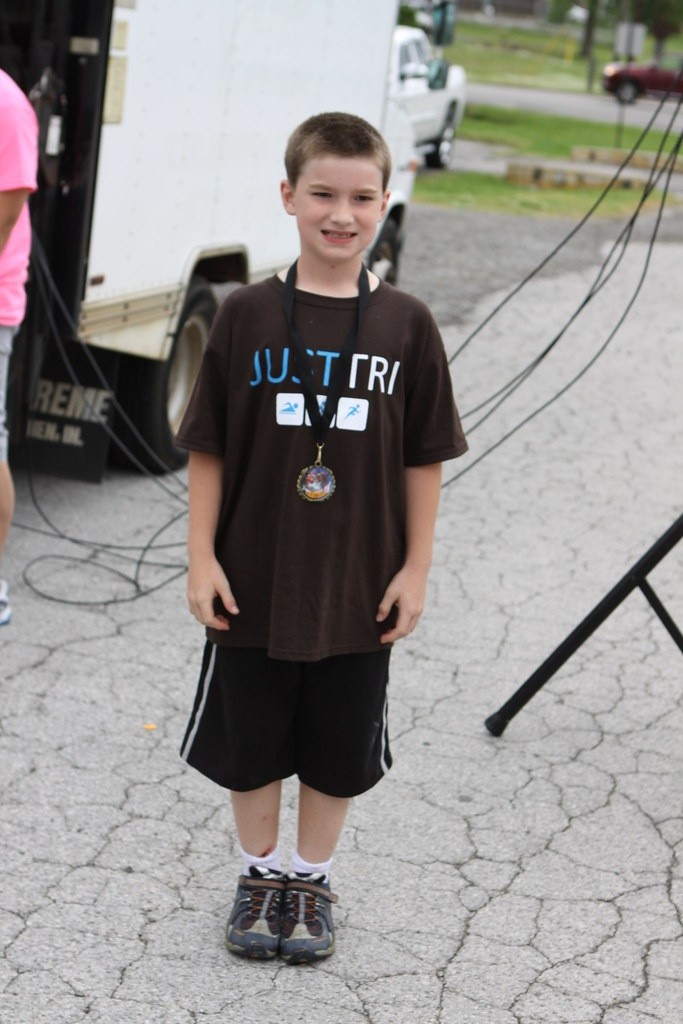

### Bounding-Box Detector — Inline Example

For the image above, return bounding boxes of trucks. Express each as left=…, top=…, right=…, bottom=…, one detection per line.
left=0, top=0, right=457, bottom=486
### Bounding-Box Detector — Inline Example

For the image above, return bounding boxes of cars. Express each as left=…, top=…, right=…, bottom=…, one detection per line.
left=398, top=1, right=454, bottom=44
left=602, top=52, right=683, bottom=105
left=393, top=24, right=470, bottom=172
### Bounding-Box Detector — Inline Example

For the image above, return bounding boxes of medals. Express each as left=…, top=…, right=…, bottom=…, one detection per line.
left=296, top=464, right=336, bottom=503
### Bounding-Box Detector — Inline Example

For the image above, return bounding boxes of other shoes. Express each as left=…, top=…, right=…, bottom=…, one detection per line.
left=0, top=577, right=11, bottom=625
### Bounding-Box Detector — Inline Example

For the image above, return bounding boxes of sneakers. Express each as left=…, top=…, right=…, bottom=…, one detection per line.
left=280, top=871, right=339, bottom=963
left=223, top=864, right=285, bottom=957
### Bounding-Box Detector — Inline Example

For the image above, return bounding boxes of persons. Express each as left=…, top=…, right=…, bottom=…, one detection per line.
left=179, top=111, right=468, bottom=961
left=0, top=61, right=39, bottom=628
